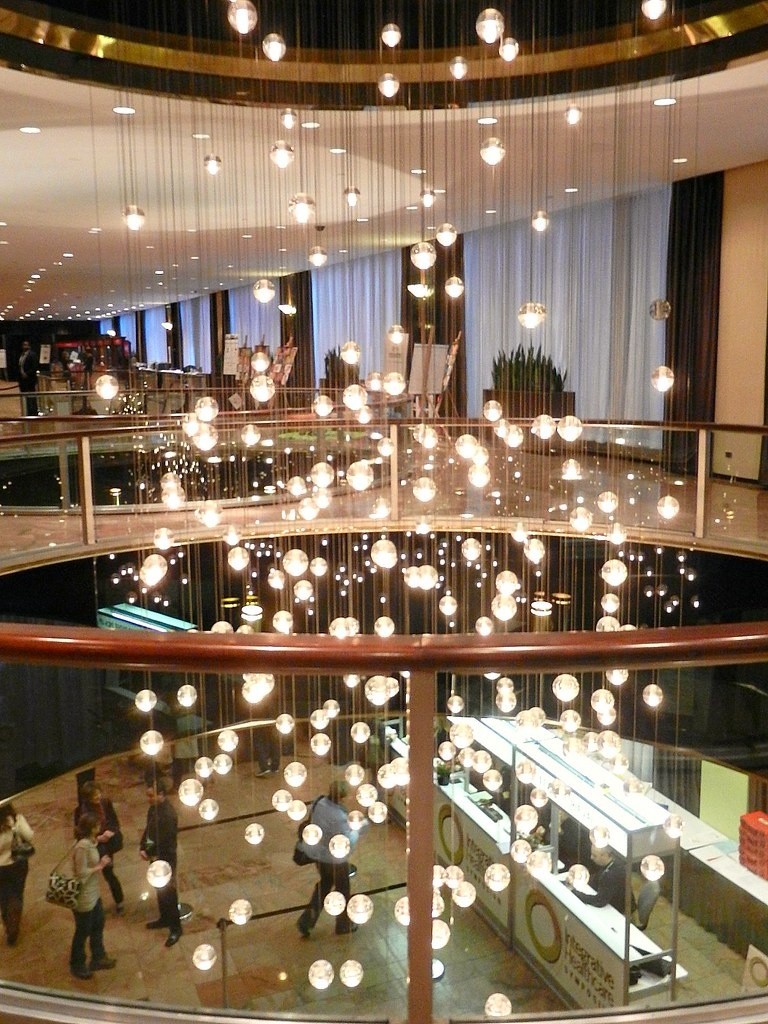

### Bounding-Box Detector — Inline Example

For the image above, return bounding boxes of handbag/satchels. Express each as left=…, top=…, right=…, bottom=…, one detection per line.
left=293, top=821, right=315, bottom=866
left=104, top=831, right=124, bottom=854
left=44, top=874, right=84, bottom=910
left=11, top=814, right=35, bottom=855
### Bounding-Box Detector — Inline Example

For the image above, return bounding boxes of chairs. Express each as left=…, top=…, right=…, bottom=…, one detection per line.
left=630, top=880, right=662, bottom=932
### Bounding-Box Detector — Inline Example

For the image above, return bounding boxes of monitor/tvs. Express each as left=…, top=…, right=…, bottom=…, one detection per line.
left=468, top=795, right=498, bottom=823
left=630, top=949, right=672, bottom=966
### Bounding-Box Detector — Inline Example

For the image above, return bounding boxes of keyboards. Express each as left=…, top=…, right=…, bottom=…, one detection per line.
left=489, top=808, right=503, bottom=820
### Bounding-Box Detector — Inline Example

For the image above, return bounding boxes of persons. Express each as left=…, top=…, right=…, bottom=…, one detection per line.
left=84, top=346, right=93, bottom=389
left=0, top=803, right=33, bottom=946
left=296, top=781, right=368, bottom=936
left=254, top=726, right=281, bottom=777
left=143, top=744, right=173, bottom=789
left=538, top=810, right=578, bottom=873
left=18, top=341, right=38, bottom=416
left=568, top=844, right=643, bottom=927
left=139, top=780, right=183, bottom=947
left=74, top=781, right=124, bottom=915
left=70, top=813, right=117, bottom=978
left=166, top=737, right=199, bottom=794
left=62, top=351, right=69, bottom=370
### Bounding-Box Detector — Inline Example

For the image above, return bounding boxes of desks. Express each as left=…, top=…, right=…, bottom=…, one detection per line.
left=452, top=776, right=768, bottom=1002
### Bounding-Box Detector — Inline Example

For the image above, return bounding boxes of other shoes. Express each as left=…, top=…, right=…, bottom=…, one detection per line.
left=117, top=906, right=125, bottom=915
left=166, top=929, right=185, bottom=947
left=90, top=955, right=117, bottom=970
left=294, top=921, right=314, bottom=936
left=255, top=768, right=271, bottom=778
left=147, top=919, right=169, bottom=928
left=269, top=763, right=280, bottom=774
left=335, top=922, right=359, bottom=934
left=7, top=935, right=18, bottom=946
left=70, top=967, right=95, bottom=979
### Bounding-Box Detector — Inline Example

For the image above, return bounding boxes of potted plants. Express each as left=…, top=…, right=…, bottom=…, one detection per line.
left=484, top=343, right=576, bottom=454
